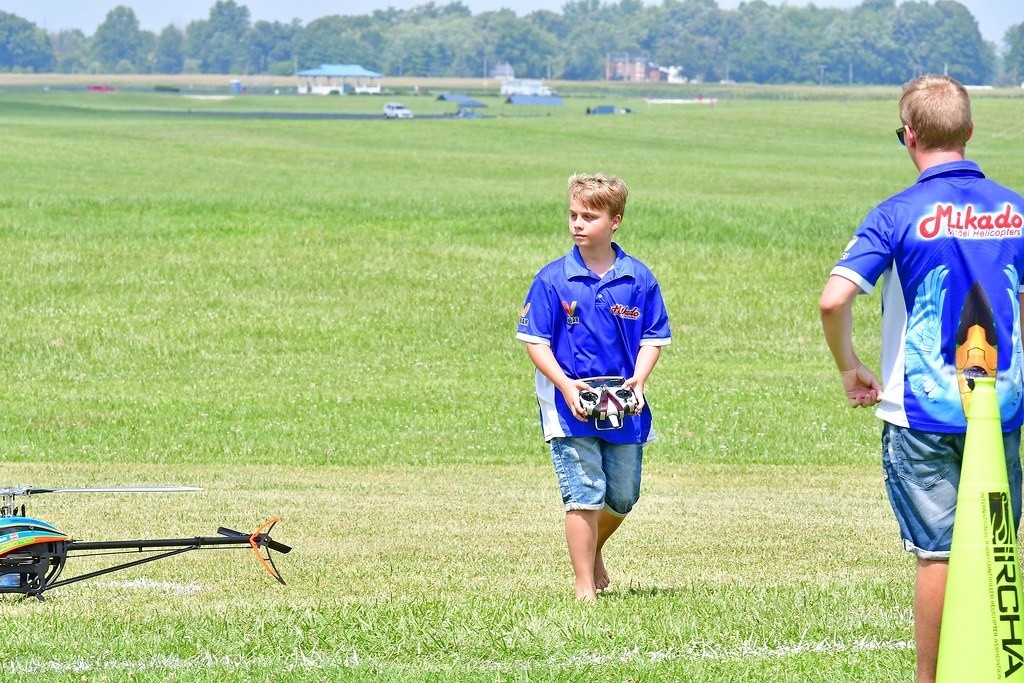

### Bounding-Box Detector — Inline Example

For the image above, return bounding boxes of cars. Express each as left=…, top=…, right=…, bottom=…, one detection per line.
left=383, top=103, right=413, bottom=120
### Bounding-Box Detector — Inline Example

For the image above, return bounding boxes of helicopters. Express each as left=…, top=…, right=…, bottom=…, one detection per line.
left=0, top=483, right=292, bottom=605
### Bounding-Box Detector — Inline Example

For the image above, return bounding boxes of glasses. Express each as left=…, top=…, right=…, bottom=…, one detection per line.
left=896, top=127, right=918, bottom=146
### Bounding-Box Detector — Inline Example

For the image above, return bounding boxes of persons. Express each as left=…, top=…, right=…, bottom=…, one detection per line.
left=818, top=72, right=1024, bottom=683
left=514, top=172, right=672, bottom=603
